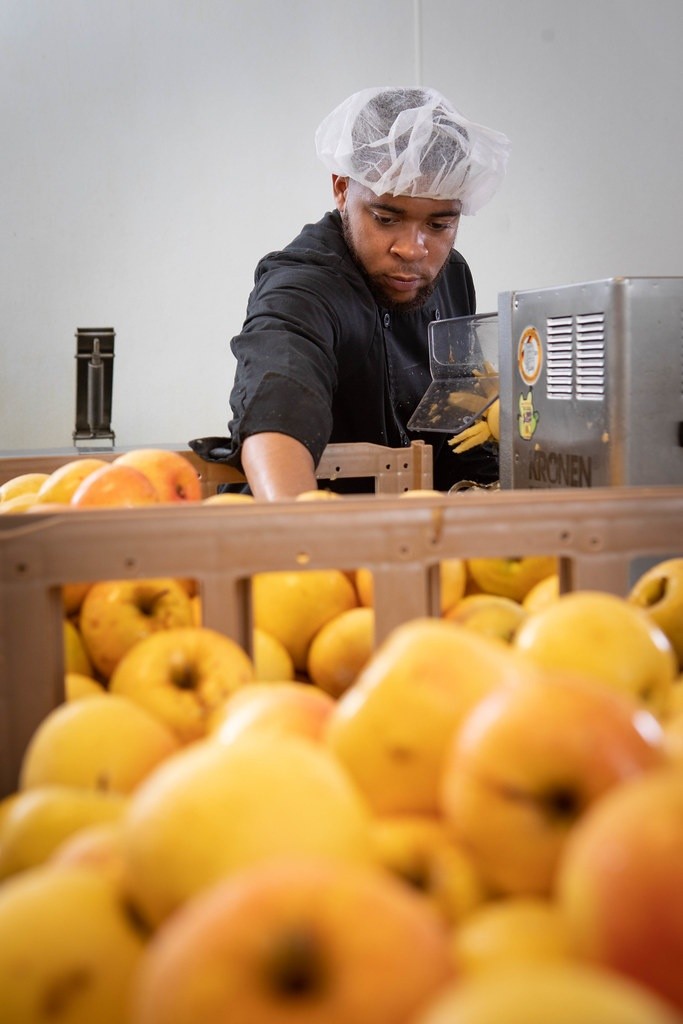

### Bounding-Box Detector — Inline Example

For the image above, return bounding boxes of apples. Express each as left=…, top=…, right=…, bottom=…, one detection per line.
left=448, top=361, right=500, bottom=453
left=0, top=447, right=683, bottom=1024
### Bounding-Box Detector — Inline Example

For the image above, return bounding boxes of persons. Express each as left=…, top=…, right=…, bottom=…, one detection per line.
left=187, top=84, right=500, bottom=503
